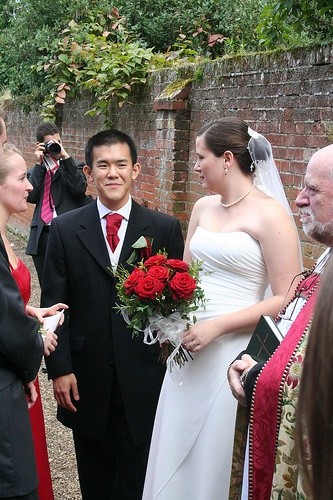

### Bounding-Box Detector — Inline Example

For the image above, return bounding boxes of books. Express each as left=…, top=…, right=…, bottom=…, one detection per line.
left=244, top=315, right=285, bottom=363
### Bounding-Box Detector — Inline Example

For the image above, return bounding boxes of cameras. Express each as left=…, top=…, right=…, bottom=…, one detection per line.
left=40, top=140, right=62, bottom=157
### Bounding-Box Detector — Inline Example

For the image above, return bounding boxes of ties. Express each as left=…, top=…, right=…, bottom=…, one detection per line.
left=104, top=212, right=124, bottom=253
left=41, top=157, right=58, bottom=223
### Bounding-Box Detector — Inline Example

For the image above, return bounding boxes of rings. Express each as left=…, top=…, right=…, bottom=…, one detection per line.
left=193, top=340, right=196, bottom=347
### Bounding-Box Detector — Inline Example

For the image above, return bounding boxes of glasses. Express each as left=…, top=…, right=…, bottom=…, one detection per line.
left=277, top=271, right=317, bottom=321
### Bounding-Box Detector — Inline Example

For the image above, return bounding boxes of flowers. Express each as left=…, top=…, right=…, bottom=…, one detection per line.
left=125, top=235, right=155, bottom=266
left=109, top=251, right=213, bottom=364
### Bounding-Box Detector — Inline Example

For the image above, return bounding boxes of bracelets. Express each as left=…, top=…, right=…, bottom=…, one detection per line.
left=238, top=363, right=257, bottom=388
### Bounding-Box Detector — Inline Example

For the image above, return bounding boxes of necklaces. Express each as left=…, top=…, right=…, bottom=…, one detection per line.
left=220, top=184, right=254, bottom=207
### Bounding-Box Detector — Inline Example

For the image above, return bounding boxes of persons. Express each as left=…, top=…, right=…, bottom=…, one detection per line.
left=141, top=117, right=303, bottom=500
left=0, top=143, right=69, bottom=500
left=227, top=143, right=333, bottom=500
left=40, top=129, right=185, bottom=500
left=25, top=122, right=88, bottom=285
left=0, top=117, right=44, bottom=500
